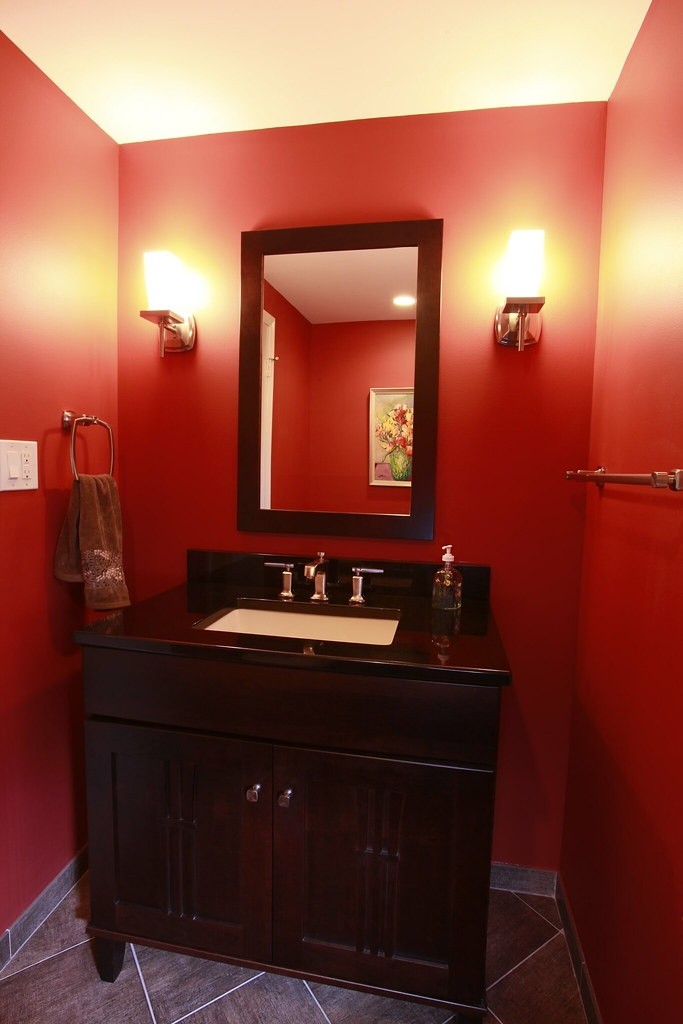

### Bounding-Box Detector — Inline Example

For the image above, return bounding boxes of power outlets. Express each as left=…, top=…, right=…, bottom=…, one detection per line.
left=0, top=439, right=39, bottom=491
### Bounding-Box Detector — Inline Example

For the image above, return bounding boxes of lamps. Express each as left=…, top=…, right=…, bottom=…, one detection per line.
left=493, top=230, right=553, bottom=355
left=136, top=248, right=201, bottom=361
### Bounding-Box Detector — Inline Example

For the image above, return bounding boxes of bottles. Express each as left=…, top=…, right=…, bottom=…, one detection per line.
left=432, top=545, right=463, bottom=610
left=432, top=608, right=462, bottom=665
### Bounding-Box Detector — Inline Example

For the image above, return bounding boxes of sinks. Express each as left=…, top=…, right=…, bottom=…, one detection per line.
left=199, top=595, right=405, bottom=647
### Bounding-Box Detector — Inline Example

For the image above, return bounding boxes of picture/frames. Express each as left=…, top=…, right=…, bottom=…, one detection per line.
left=368, top=387, right=414, bottom=488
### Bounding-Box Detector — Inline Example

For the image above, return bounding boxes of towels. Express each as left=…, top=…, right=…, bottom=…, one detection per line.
left=53, top=473, right=133, bottom=612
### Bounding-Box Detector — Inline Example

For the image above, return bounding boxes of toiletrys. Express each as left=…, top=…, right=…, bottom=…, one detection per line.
left=431, top=543, right=463, bottom=610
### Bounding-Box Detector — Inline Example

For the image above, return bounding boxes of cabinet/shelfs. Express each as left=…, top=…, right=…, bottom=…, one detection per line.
left=80, top=645, right=506, bottom=1024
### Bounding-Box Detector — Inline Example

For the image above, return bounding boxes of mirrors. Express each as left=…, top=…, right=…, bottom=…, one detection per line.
left=235, top=217, right=446, bottom=541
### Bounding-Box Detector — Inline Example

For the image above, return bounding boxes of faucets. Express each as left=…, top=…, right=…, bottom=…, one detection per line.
left=303, top=550, right=330, bottom=601
